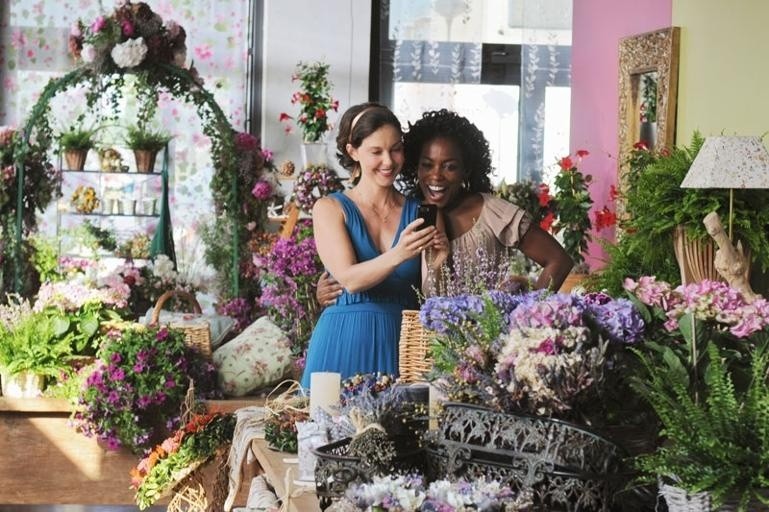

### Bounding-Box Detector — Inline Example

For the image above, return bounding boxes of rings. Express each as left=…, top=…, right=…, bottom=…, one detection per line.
left=418, top=247, right=423, bottom=250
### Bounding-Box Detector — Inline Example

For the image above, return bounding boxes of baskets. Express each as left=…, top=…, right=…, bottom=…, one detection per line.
left=149, top=290, right=213, bottom=358
left=397, top=309, right=435, bottom=383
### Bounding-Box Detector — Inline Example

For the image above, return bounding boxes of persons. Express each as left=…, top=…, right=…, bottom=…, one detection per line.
left=316, top=110, right=575, bottom=306
left=300, top=103, right=451, bottom=390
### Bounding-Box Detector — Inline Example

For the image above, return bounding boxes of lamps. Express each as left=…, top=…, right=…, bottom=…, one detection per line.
left=679, top=134, right=769, bottom=248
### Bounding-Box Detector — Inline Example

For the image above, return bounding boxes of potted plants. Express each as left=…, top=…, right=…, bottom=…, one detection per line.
left=123, top=122, right=177, bottom=173
left=1, top=312, right=80, bottom=398
left=57, top=122, right=115, bottom=171
left=628, top=343, right=769, bottom=512
left=649, top=133, right=769, bottom=286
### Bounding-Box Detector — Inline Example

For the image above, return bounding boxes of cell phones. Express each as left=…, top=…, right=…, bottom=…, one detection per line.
left=416, top=203, right=439, bottom=231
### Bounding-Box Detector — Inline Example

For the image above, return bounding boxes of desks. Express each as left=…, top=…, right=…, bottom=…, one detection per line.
left=245, top=420, right=325, bottom=511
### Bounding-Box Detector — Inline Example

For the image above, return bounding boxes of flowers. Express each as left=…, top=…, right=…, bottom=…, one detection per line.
left=280, top=62, right=341, bottom=143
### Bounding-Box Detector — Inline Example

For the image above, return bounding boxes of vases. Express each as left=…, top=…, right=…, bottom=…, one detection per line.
left=299, top=142, right=328, bottom=173
